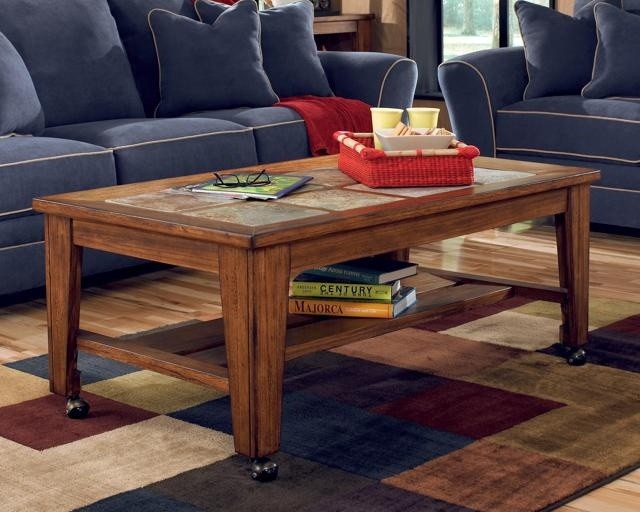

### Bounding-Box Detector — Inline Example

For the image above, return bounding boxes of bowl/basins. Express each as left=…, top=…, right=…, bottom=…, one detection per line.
left=373, top=127, right=456, bottom=150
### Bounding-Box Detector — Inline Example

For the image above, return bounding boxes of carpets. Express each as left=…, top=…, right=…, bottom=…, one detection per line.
left=0, top=294, right=640, bottom=511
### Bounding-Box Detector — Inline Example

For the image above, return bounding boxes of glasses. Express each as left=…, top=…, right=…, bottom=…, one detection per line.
left=211, top=169, right=271, bottom=187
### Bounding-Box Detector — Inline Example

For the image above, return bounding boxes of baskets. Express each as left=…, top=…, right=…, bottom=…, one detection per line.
left=332, top=129, right=480, bottom=190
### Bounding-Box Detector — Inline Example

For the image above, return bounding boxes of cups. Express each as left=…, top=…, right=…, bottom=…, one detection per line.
left=369, top=105, right=404, bottom=151
left=406, top=106, right=440, bottom=129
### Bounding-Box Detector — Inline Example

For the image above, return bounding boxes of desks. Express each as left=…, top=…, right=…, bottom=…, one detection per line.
left=313, top=13, right=376, bottom=53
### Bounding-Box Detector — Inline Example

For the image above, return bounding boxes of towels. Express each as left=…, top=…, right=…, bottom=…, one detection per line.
left=272, top=94, right=375, bottom=157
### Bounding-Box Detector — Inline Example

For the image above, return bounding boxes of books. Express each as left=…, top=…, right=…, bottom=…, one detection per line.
left=301, top=255, right=419, bottom=285
left=290, top=271, right=403, bottom=301
left=159, top=169, right=314, bottom=201
left=286, top=286, right=419, bottom=319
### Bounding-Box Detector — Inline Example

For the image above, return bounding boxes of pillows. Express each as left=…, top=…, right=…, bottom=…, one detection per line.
left=147, top=8, right=280, bottom=119
left=0, top=0, right=147, bottom=128
left=108, top=0, right=200, bottom=116
left=0, top=31, right=46, bottom=138
left=194, top=0, right=335, bottom=98
left=513, top=0, right=597, bottom=102
left=578, top=2, right=640, bottom=100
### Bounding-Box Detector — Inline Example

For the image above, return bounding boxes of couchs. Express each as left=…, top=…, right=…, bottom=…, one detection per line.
left=437, top=0, right=640, bottom=229
left=0, top=51, right=418, bottom=294
